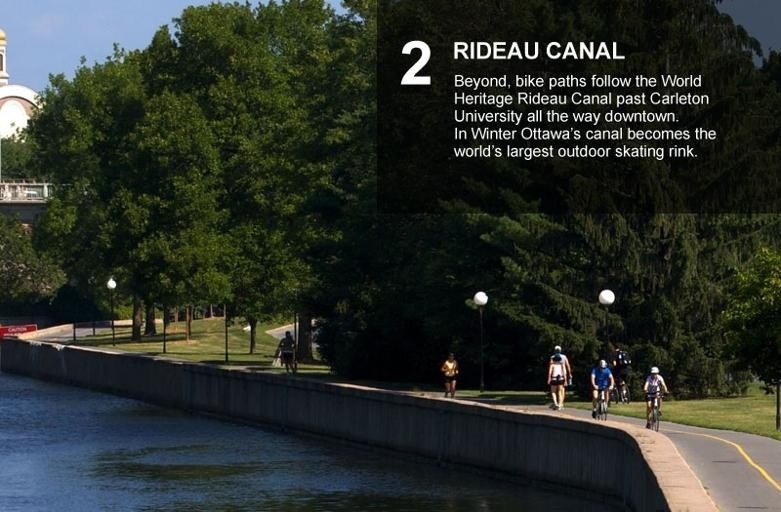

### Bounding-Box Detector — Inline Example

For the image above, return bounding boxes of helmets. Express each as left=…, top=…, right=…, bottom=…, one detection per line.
left=649, top=366, right=660, bottom=374
left=599, top=359, right=607, bottom=369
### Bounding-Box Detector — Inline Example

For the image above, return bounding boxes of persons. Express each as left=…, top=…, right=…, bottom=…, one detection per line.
left=547, top=355, right=568, bottom=410
left=550, top=345, right=573, bottom=408
left=643, top=366, right=670, bottom=429
left=279, top=332, right=297, bottom=369
left=590, top=359, right=616, bottom=419
left=610, top=343, right=632, bottom=403
left=282, top=335, right=296, bottom=375
left=441, top=351, right=460, bottom=399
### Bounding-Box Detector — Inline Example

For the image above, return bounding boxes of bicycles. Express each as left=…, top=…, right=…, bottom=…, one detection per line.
left=590, top=377, right=662, bottom=433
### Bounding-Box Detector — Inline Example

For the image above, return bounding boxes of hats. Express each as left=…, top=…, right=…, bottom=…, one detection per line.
left=554, top=345, right=562, bottom=351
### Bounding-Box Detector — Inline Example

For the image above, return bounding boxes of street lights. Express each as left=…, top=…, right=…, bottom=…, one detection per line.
left=105, top=277, right=119, bottom=347
left=597, top=287, right=615, bottom=367
left=473, top=290, right=489, bottom=393
